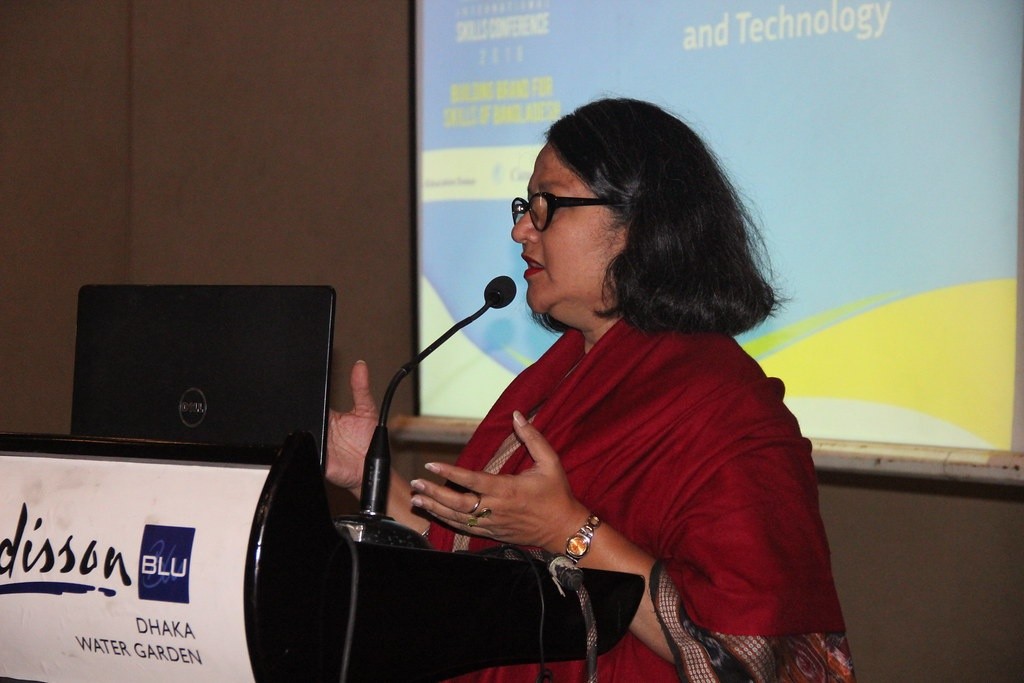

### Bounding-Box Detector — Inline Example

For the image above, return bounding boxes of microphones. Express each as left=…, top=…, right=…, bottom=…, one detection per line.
left=338, top=276, right=517, bottom=548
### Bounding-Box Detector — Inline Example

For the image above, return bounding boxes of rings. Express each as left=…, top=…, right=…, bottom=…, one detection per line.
left=468, top=495, right=492, bottom=527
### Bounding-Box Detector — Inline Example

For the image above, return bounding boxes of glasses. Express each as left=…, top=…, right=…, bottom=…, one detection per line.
left=511, top=191, right=622, bottom=233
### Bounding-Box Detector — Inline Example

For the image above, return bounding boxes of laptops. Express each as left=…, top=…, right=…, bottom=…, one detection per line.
left=69, top=284, right=337, bottom=474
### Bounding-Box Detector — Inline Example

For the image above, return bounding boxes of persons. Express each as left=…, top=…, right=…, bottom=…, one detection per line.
left=325, top=102, right=859, bottom=683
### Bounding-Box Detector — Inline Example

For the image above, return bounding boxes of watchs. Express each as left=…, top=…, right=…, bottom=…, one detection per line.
left=567, top=512, right=602, bottom=564
